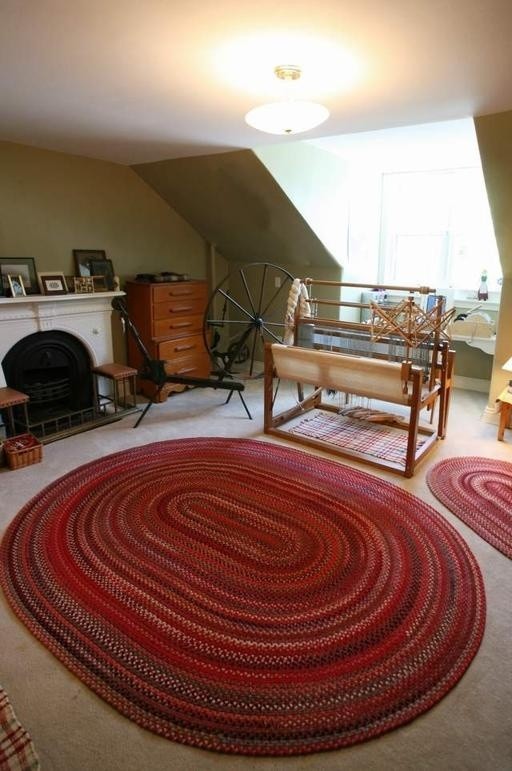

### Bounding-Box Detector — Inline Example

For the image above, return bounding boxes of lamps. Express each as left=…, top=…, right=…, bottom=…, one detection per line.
left=245, top=60, right=330, bottom=135
left=503, top=353, right=512, bottom=395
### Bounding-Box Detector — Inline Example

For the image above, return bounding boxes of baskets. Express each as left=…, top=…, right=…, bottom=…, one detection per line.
left=2, top=433, right=43, bottom=470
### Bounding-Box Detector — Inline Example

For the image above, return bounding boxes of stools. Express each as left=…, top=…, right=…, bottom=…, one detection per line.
left=91, top=362, right=140, bottom=415
left=0, top=386, right=33, bottom=442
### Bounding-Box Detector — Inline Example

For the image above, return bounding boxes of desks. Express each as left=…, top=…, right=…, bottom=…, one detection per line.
left=497, top=387, right=512, bottom=442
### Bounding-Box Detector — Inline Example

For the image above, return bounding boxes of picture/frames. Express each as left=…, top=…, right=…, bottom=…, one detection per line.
left=0, top=247, right=118, bottom=295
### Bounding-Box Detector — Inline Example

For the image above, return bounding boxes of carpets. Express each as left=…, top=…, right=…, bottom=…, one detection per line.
left=427, top=458, right=512, bottom=558
left=1, top=436, right=486, bottom=755
left=1, top=688, right=40, bottom=771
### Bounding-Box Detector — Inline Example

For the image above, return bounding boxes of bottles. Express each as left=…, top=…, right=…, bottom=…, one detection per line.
left=476, top=269, right=489, bottom=300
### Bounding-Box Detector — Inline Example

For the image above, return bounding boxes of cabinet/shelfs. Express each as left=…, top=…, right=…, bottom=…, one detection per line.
left=129, top=282, right=213, bottom=402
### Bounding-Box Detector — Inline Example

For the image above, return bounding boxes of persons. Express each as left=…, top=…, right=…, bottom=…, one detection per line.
left=75, top=278, right=92, bottom=292
left=11, top=277, right=23, bottom=296
